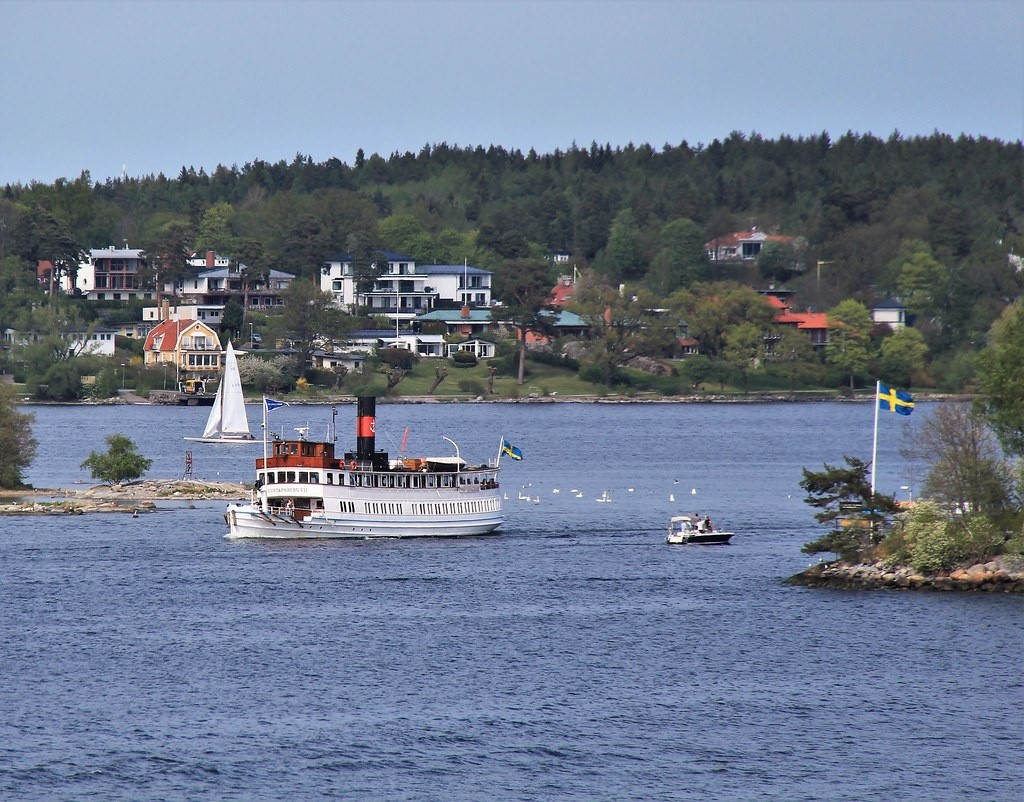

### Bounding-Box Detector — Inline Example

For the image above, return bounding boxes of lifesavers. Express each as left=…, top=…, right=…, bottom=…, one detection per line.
left=339, top=459, right=345, bottom=468
left=350, top=461, right=358, bottom=471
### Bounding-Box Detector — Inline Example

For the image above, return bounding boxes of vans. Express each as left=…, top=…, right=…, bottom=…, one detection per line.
left=182, top=378, right=206, bottom=394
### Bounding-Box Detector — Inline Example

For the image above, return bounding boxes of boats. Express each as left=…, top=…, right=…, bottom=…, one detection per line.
left=221, top=393, right=505, bottom=541
left=665, top=515, right=735, bottom=545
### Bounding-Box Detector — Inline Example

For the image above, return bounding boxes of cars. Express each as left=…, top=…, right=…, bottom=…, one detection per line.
left=252, top=334, right=262, bottom=349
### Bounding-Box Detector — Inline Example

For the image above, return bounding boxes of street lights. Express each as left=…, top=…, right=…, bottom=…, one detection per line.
left=162, top=364, right=168, bottom=390
left=120, top=364, right=125, bottom=389
left=249, top=323, right=253, bottom=348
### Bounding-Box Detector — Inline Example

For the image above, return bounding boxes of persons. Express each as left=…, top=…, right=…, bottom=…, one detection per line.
left=286, top=500, right=295, bottom=516
left=681, top=513, right=713, bottom=533
left=243, top=433, right=255, bottom=439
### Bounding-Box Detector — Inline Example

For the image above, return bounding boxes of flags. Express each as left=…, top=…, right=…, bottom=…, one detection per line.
left=265, top=398, right=290, bottom=412
left=501, top=438, right=523, bottom=461
left=879, top=384, right=915, bottom=415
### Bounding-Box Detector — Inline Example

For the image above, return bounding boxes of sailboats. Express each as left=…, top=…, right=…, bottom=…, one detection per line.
left=181, top=337, right=275, bottom=444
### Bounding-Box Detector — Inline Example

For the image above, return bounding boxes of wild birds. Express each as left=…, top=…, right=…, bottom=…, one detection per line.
left=502, top=479, right=700, bottom=503
